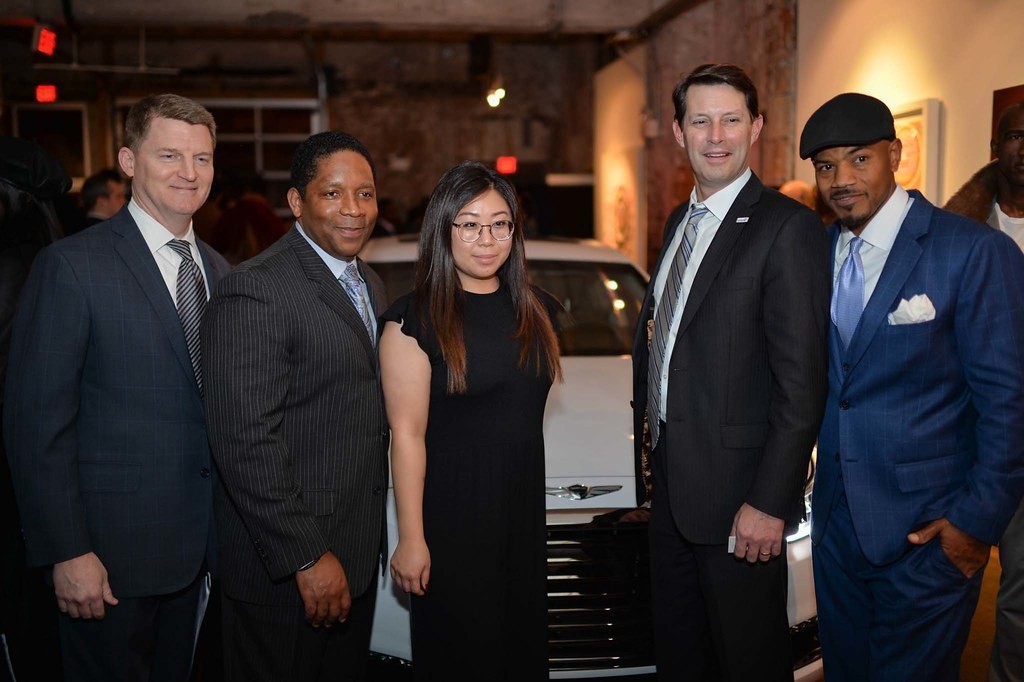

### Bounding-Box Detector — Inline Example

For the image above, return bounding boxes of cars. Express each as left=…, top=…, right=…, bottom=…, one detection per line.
left=339, top=227, right=832, bottom=682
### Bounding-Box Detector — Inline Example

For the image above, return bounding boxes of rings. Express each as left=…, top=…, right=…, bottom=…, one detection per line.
left=761, top=552, right=771, bottom=556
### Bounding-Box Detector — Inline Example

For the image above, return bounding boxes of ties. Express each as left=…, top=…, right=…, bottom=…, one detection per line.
left=166, top=240, right=205, bottom=402
left=831, top=237, right=866, bottom=348
left=647, top=203, right=709, bottom=450
left=339, top=264, right=374, bottom=350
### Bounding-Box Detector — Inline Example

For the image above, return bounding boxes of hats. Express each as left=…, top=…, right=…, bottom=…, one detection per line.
left=799, top=93, right=896, bottom=160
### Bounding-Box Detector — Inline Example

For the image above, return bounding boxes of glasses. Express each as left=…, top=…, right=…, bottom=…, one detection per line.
left=453, top=220, right=514, bottom=243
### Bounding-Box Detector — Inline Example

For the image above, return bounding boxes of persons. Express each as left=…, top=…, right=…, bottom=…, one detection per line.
left=376, top=159, right=577, bottom=682
left=798, top=90, right=1024, bottom=681
left=197, top=131, right=393, bottom=682
left=3, top=92, right=235, bottom=682
left=938, top=99, right=1024, bottom=682
left=1, top=131, right=539, bottom=317
left=630, top=62, right=832, bottom=681
